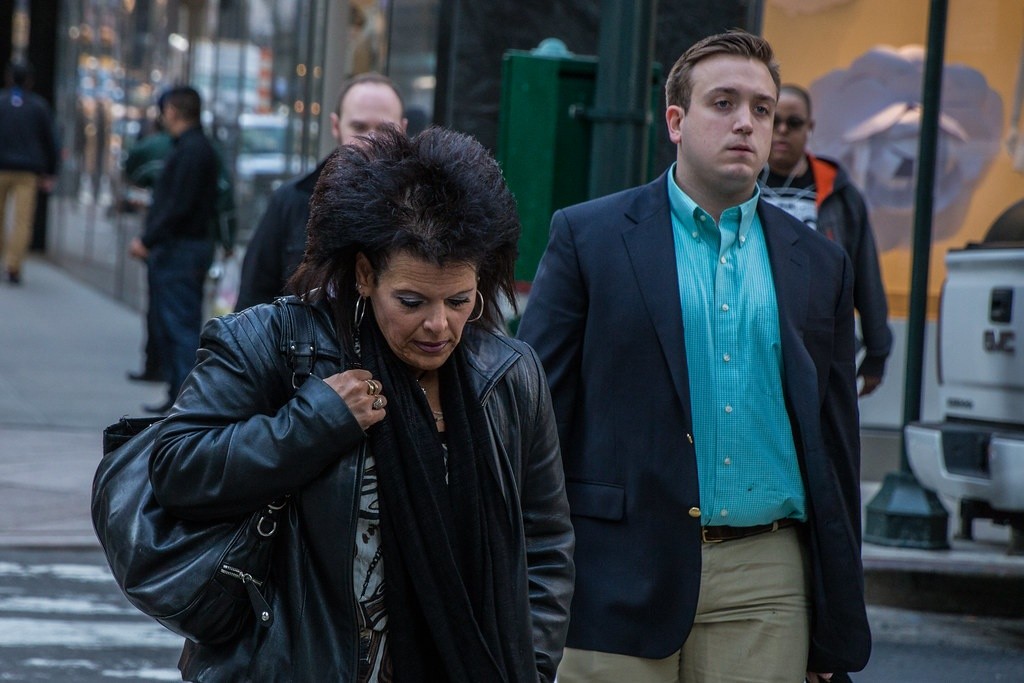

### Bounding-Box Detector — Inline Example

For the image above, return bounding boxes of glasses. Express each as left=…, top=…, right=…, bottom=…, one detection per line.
left=770, top=115, right=809, bottom=133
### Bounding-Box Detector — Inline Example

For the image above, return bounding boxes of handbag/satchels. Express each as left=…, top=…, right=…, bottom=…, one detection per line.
left=88, top=293, right=308, bottom=654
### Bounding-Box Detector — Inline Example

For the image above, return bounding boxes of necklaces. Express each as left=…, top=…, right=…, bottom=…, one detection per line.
left=422, top=387, right=443, bottom=422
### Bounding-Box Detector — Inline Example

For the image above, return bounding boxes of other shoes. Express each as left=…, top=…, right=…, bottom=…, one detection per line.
left=140, top=394, right=176, bottom=414
left=125, top=363, right=171, bottom=385
left=7, top=270, right=21, bottom=285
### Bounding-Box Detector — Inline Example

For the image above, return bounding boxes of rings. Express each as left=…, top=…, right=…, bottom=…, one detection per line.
left=373, top=395, right=383, bottom=408
left=364, top=379, right=378, bottom=394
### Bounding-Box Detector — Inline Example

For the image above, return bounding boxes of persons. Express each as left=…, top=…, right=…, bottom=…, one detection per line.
left=150, top=127, right=575, bottom=683
left=125, top=85, right=230, bottom=416
left=0, top=61, right=63, bottom=283
left=758, top=84, right=893, bottom=396
left=516, top=34, right=882, bottom=682
left=231, top=71, right=407, bottom=312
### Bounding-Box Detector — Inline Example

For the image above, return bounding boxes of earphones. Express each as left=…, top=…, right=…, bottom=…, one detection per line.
left=807, top=130, right=812, bottom=139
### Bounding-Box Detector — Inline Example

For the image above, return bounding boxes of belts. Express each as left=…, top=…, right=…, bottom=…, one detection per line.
left=693, top=517, right=796, bottom=543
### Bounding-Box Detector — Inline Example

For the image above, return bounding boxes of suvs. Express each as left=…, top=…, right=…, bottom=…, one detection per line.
left=228, top=112, right=319, bottom=222
